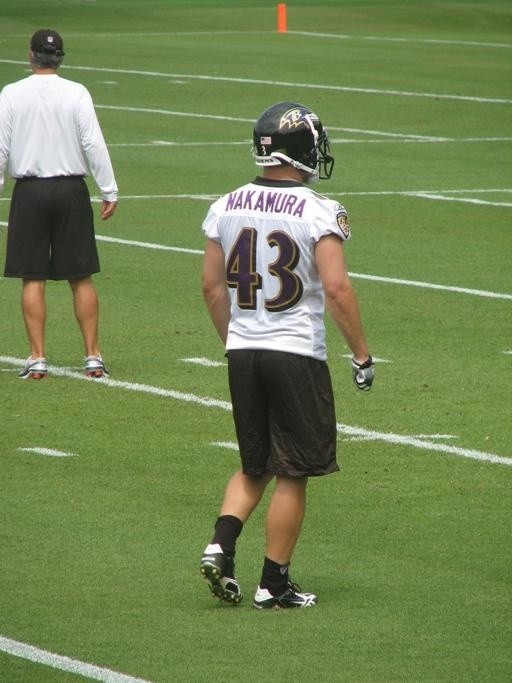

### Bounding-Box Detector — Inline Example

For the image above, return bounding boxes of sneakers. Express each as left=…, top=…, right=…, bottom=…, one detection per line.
left=254, top=578, right=317, bottom=610
left=200, top=542, right=243, bottom=605
left=17, top=355, right=48, bottom=380
left=84, top=356, right=108, bottom=377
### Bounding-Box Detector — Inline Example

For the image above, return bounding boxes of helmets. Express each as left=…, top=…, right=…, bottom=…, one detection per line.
left=251, top=101, right=335, bottom=184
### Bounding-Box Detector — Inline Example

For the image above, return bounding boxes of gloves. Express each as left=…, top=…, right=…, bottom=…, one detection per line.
left=351, top=355, right=375, bottom=391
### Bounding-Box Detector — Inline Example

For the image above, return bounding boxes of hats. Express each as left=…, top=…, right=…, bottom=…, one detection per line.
left=29, top=28, right=65, bottom=58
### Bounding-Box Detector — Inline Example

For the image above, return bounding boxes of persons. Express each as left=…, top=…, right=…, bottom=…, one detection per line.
left=198, top=102, right=372, bottom=610
left=0, top=29, right=118, bottom=381
